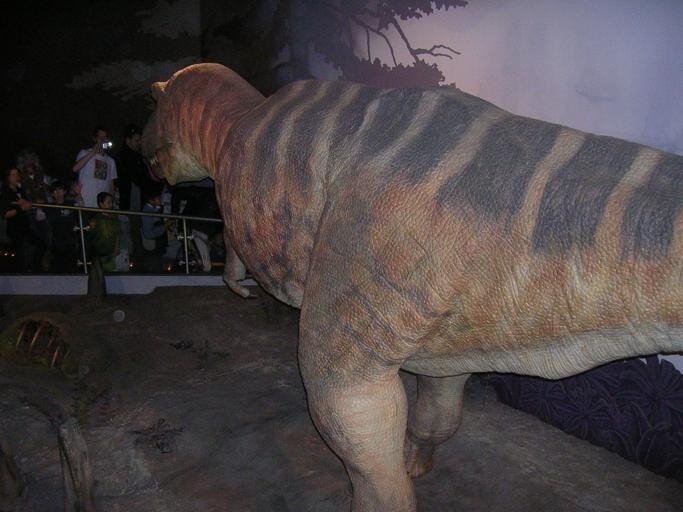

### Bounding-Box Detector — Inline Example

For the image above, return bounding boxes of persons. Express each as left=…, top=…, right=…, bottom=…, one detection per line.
left=0, top=128, right=171, bottom=273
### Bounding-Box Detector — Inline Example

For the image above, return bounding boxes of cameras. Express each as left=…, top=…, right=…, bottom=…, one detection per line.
left=101, top=140, right=113, bottom=150
